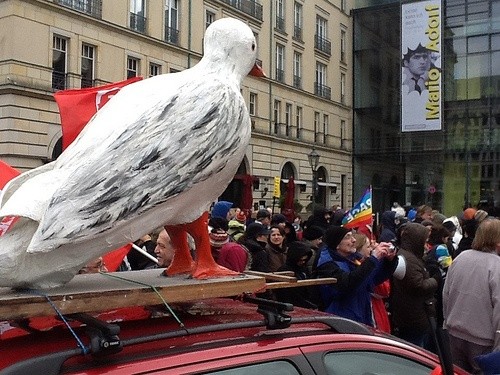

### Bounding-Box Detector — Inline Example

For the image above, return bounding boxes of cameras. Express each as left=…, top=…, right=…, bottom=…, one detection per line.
left=387, top=243, right=395, bottom=253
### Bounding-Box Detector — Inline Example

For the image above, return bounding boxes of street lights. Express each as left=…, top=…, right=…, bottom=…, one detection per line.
left=307, top=144, right=321, bottom=203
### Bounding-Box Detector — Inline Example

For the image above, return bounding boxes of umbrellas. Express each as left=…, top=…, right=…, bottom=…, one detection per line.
left=283, top=176, right=295, bottom=222
left=242, top=174, right=253, bottom=208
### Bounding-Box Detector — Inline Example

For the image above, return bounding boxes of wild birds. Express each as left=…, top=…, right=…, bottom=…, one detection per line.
left=0, top=15, right=266, bottom=289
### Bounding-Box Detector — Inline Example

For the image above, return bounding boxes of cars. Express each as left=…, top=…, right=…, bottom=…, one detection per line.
left=0, top=294, right=473, bottom=375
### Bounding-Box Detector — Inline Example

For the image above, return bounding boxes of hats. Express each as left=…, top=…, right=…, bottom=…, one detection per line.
left=464, top=208, right=476, bottom=220
left=208, top=227, right=230, bottom=247
left=302, top=227, right=326, bottom=241
left=322, top=226, right=351, bottom=250
left=256, top=209, right=270, bottom=220
left=236, top=211, right=246, bottom=221
left=474, top=210, right=488, bottom=222
left=391, top=202, right=406, bottom=217
left=246, top=223, right=273, bottom=237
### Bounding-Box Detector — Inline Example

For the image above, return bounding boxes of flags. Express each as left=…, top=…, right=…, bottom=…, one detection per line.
left=53, top=76, right=143, bottom=154
left=0, top=160, right=21, bottom=236
left=341, top=186, right=372, bottom=227
left=101, top=242, right=133, bottom=272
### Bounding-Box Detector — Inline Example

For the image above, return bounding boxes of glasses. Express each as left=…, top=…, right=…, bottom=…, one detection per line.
left=82, top=260, right=105, bottom=269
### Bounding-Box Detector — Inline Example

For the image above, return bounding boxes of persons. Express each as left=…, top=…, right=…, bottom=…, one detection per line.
left=402, top=42, right=431, bottom=95
left=79, top=202, right=500, bottom=375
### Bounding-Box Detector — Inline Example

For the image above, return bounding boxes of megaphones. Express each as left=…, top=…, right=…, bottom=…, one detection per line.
left=292, top=223, right=300, bottom=231
